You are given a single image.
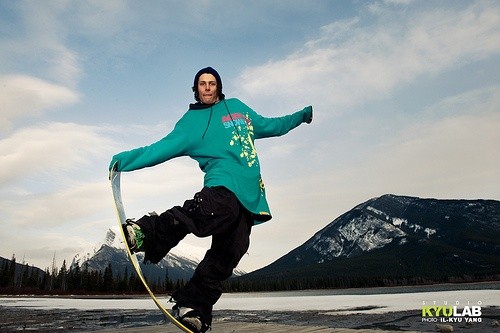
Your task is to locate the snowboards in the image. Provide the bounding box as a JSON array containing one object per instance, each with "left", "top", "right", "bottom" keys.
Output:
[{"left": 110, "top": 161, "right": 195, "bottom": 333}]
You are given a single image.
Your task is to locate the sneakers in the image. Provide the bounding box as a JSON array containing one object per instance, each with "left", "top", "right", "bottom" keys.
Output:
[
  {"left": 172, "top": 305, "right": 202, "bottom": 332},
  {"left": 121, "top": 218, "right": 146, "bottom": 255}
]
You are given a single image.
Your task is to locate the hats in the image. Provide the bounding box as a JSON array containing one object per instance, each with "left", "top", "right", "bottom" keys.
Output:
[{"left": 192, "top": 66, "right": 222, "bottom": 102}]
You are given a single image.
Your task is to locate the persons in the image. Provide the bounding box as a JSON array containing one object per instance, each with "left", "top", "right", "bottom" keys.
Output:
[{"left": 108, "top": 66, "right": 313, "bottom": 332}]
